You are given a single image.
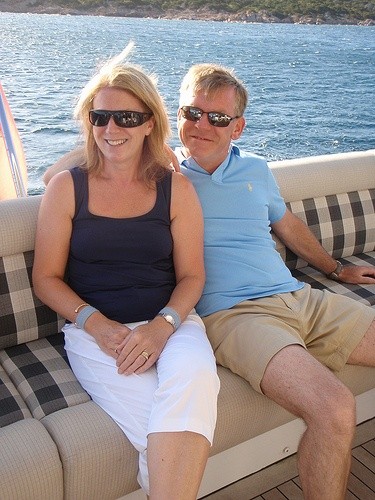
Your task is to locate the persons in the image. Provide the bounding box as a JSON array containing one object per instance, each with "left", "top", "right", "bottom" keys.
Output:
[
  {"left": 43, "top": 62, "right": 375, "bottom": 500},
  {"left": 33, "top": 65, "right": 220, "bottom": 500}
]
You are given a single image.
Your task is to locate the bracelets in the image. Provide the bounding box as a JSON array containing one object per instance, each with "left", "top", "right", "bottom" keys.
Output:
[
  {"left": 72, "top": 303, "right": 98, "bottom": 328},
  {"left": 159, "top": 306, "right": 181, "bottom": 330}
]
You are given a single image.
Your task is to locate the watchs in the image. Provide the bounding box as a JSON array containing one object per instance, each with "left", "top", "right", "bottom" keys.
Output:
[
  {"left": 157, "top": 312, "right": 176, "bottom": 332},
  {"left": 330, "top": 259, "right": 342, "bottom": 278}
]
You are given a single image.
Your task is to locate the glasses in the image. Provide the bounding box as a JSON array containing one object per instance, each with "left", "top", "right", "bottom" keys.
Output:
[
  {"left": 89, "top": 110, "right": 154, "bottom": 128},
  {"left": 181, "top": 106, "right": 242, "bottom": 127}
]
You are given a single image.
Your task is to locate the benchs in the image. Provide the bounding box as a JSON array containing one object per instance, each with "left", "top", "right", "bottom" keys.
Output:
[{"left": 0, "top": 148, "right": 375, "bottom": 500}]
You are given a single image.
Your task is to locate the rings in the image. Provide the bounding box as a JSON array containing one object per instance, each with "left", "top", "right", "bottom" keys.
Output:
[
  {"left": 141, "top": 351, "right": 148, "bottom": 359},
  {"left": 115, "top": 345, "right": 120, "bottom": 352}
]
